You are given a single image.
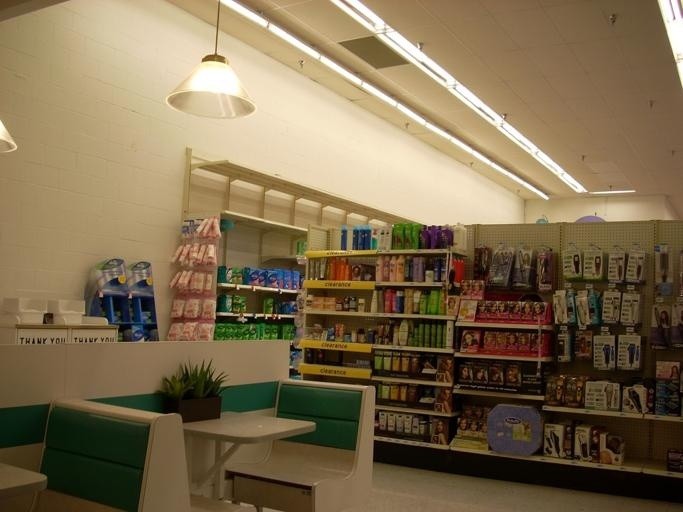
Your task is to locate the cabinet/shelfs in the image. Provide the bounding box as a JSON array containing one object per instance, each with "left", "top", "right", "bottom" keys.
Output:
[
  {"left": 183, "top": 147, "right": 424, "bottom": 343},
  {"left": 297, "top": 225, "right": 682, "bottom": 483}
]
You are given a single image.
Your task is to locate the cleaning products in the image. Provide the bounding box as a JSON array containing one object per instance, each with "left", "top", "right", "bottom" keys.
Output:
[{"left": 304, "top": 222, "right": 468, "bottom": 438}]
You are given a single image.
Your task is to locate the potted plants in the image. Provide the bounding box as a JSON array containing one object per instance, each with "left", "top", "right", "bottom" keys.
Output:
[{"left": 153, "top": 357, "right": 229, "bottom": 423}]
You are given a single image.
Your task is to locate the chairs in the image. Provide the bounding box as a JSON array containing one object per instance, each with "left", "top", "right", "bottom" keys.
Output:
[{"left": 30, "top": 378, "right": 376, "bottom": 511}]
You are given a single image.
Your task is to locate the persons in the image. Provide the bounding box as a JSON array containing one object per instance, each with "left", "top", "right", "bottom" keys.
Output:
[
  {"left": 428, "top": 281, "right": 544, "bottom": 445},
  {"left": 668, "top": 365, "right": 679, "bottom": 379},
  {"left": 660, "top": 310, "right": 669, "bottom": 326}
]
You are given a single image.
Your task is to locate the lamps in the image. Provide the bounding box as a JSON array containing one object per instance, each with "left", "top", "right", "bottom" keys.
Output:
[{"left": 164, "top": 0, "right": 256, "bottom": 120}]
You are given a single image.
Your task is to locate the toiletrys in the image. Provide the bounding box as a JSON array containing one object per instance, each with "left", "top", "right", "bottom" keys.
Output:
[
  {"left": 412, "top": 323, "right": 448, "bottom": 348},
  {"left": 303, "top": 294, "right": 399, "bottom": 346}
]
